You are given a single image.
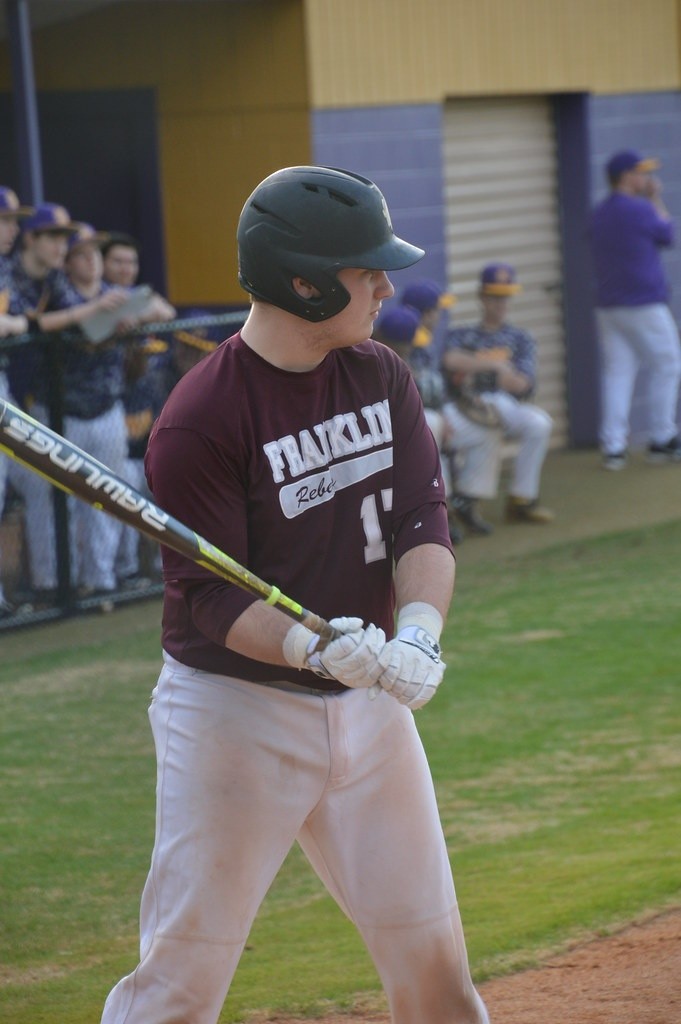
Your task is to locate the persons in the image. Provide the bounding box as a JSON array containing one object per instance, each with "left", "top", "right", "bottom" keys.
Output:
[
  {"left": 0, "top": 186, "right": 218, "bottom": 619},
  {"left": 441, "top": 263, "right": 554, "bottom": 534},
  {"left": 584, "top": 152, "right": 681, "bottom": 472},
  {"left": 97, "top": 166, "right": 491, "bottom": 1023},
  {"left": 375, "top": 263, "right": 500, "bottom": 543}
]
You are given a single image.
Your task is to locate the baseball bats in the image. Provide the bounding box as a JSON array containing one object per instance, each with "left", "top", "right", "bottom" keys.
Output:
[{"left": 0, "top": 398, "right": 342, "bottom": 643}]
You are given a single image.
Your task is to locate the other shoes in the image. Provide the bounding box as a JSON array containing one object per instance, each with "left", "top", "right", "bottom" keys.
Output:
[
  {"left": 447, "top": 494, "right": 495, "bottom": 535},
  {"left": 0, "top": 570, "right": 151, "bottom": 615},
  {"left": 647, "top": 437, "right": 681, "bottom": 461},
  {"left": 604, "top": 448, "right": 630, "bottom": 470},
  {"left": 449, "top": 526, "right": 465, "bottom": 545},
  {"left": 505, "top": 494, "right": 552, "bottom": 523}
]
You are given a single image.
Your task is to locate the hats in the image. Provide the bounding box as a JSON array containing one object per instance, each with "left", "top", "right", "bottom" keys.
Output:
[
  {"left": 70, "top": 221, "right": 108, "bottom": 249},
  {"left": 400, "top": 279, "right": 458, "bottom": 315},
  {"left": 478, "top": 263, "right": 522, "bottom": 297},
  {"left": 374, "top": 305, "right": 433, "bottom": 348},
  {"left": 0, "top": 188, "right": 36, "bottom": 220},
  {"left": 16, "top": 201, "right": 81, "bottom": 234}
]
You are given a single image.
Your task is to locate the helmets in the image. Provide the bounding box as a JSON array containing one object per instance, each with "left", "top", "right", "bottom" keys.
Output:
[{"left": 234, "top": 165, "right": 426, "bottom": 322}]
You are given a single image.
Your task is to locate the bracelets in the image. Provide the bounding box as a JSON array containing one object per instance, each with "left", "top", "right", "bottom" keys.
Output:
[
  {"left": 398, "top": 602, "right": 443, "bottom": 643},
  {"left": 283, "top": 623, "right": 316, "bottom": 668}
]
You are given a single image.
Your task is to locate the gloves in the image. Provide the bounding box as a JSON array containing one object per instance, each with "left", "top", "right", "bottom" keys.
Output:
[
  {"left": 368, "top": 625, "right": 447, "bottom": 710},
  {"left": 307, "top": 616, "right": 393, "bottom": 689}
]
